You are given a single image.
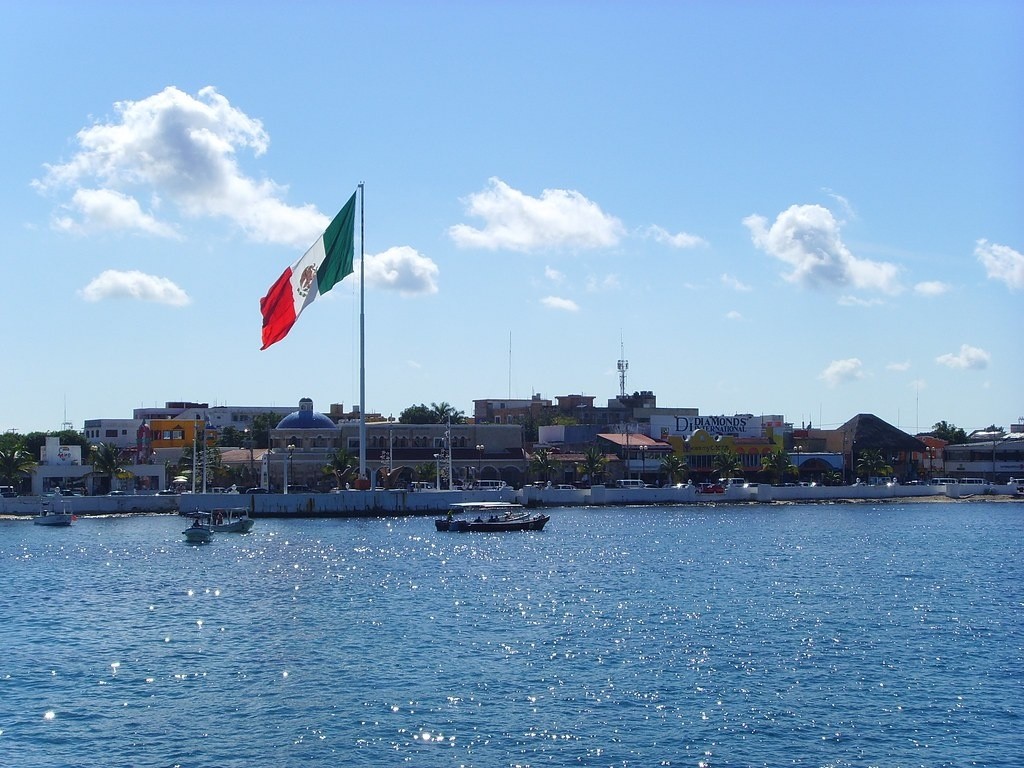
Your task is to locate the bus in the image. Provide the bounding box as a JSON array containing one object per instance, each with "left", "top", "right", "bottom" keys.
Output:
[
  {"left": 475, "top": 479, "right": 504, "bottom": 490},
  {"left": 930, "top": 477, "right": 958, "bottom": 485},
  {"left": 615, "top": 480, "right": 645, "bottom": 489},
  {"left": 960, "top": 477, "right": 988, "bottom": 485},
  {"left": 1014, "top": 479, "right": 1024, "bottom": 493},
  {"left": 717, "top": 478, "right": 744, "bottom": 488}
]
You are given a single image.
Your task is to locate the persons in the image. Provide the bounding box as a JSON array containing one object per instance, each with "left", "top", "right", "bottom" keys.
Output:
[
  {"left": 206, "top": 510, "right": 224, "bottom": 524},
  {"left": 461, "top": 481, "right": 478, "bottom": 490},
  {"left": 193, "top": 520, "right": 200, "bottom": 527},
  {"left": 416, "top": 481, "right": 421, "bottom": 492},
  {"left": 81, "top": 486, "right": 89, "bottom": 496},
  {"left": 494, "top": 515, "right": 500, "bottom": 522},
  {"left": 488, "top": 516, "right": 495, "bottom": 522},
  {"left": 446, "top": 508, "right": 453, "bottom": 522},
  {"left": 360, "top": 474, "right": 368, "bottom": 494},
  {"left": 474, "top": 516, "right": 484, "bottom": 523},
  {"left": 407, "top": 481, "right": 411, "bottom": 493}
]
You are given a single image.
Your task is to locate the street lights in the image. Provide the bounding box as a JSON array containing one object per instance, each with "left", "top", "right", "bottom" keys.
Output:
[
  {"left": 639, "top": 445, "right": 648, "bottom": 483},
  {"left": 925, "top": 446, "right": 936, "bottom": 485},
  {"left": 287, "top": 444, "right": 295, "bottom": 486},
  {"left": 794, "top": 446, "right": 803, "bottom": 485},
  {"left": 90, "top": 445, "right": 99, "bottom": 495},
  {"left": 475, "top": 444, "right": 484, "bottom": 479}
]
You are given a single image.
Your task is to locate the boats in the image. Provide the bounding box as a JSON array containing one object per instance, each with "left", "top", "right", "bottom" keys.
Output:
[
  {"left": 181, "top": 505, "right": 215, "bottom": 542},
  {"left": 434, "top": 500, "right": 552, "bottom": 531},
  {"left": 34, "top": 496, "right": 73, "bottom": 526},
  {"left": 210, "top": 507, "right": 255, "bottom": 531}
]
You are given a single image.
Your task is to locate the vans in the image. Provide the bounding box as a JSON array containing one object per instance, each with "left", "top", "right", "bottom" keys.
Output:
[
  {"left": 556, "top": 484, "right": 578, "bottom": 490},
  {"left": 0, "top": 486, "right": 14, "bottom": 498},
  {"left": 410, "top": 482, "right": 436, "bottom": 492}
]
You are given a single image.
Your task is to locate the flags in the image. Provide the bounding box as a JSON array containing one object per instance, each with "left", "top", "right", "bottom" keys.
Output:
[{"left": 258, "top": 190, "right": 356, "bottom": 350}]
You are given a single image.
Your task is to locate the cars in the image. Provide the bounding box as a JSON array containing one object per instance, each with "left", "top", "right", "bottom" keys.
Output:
[
  {"left": 662, "top": 483, "right": 725, "bottom": 494},
  {"left": 743, "top": 482, "right": 809, "bottom": 488},
  {"left": 107, "top": 491, "right": 125, "bottom": 495},
  {"left": 60, "top": 490, "right": 74, "bottom": 496},
  {"left": 640, "top": 484, "right": 660, "bottom": 488},
  {"left": 210, "top": 486, "right": 280, "bottom": 493},
  {"left": 155, "top": 490, "right": 177, "bottom": 495}
]
